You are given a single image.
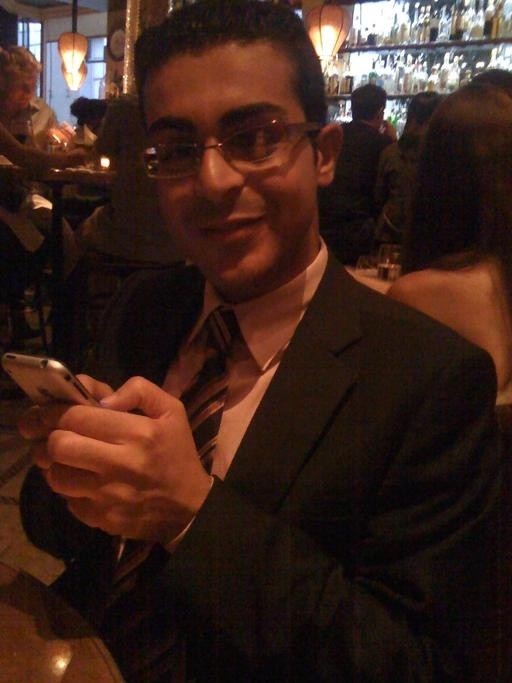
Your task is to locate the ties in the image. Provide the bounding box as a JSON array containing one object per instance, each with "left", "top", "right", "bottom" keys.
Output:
[{"left": 98, "top": 308, "right": 239, "bottom": 682}]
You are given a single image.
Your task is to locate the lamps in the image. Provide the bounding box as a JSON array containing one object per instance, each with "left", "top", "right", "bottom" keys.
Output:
[
  {"left": 305, "top": 3, "right": 353, "bottom": 73},
  {"left": 58, "top": 1, "right": 87, "bottom": 92}
]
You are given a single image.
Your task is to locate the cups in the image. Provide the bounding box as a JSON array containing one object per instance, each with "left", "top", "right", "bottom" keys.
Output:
[{"left": 376, "top": 243, "right": 402, "bottom": 280}]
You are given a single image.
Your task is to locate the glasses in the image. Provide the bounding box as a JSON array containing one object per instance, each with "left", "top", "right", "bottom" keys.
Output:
[{"left": 142, "top": 120, "right": 321, "bottom": 181}]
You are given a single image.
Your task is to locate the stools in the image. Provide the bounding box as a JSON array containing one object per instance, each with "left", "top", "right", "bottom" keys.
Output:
[{"left": 0, "top": 249, "right": 183, "bottom": 398}]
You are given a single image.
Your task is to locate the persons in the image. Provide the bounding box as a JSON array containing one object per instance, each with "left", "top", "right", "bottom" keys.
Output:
[
  {"left": 375, "top": 89, "right": 444, "bottom": 244},
  {"left": 384, "top": 80, "right": 512, "bottom": 431},
  {"left": 0, "top": 120, "right": 92, "bottom": 170},
  {"left": 468, "top": 68, "right": 512, "bottom": 100},
  {"left": 75, "top": 90, "right": 189, "bottom": 264},
  {"left": 18, "top": 0, "right": 506, "bottom": 681},
  {"left": 0, "top": 42, "right": 81, "bottom": 270},
  {"left": 318, "top": 83, "right": 399, "bottom": 264}
]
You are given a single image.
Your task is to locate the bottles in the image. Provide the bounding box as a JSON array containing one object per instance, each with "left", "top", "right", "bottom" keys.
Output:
[{"left": 332, "top": 0, "right": 512, "bottom": 137}]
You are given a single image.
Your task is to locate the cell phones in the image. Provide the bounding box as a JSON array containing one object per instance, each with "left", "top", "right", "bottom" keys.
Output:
[{"left": 1, "top": 351, "right": 104, "bottom": 407}]
